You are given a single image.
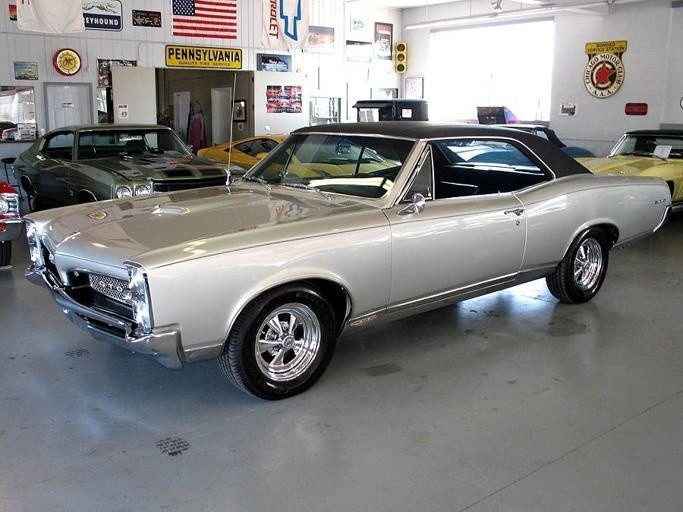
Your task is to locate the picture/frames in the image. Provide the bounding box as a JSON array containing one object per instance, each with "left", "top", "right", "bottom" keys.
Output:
[
  {"left": 349, "top": 15, "right": 366, "bottom": 34},
  {"left": 231, "top": 98, "right": 247, "bottom": 124},
  {"left": 404, "top": 78, "right": 424, "bottom": 98},
  {"left": 302, "top": 25, "right": 335, "bottom": 54},
  {"left": 373, "top": 21, "right": 394, "bottom": 60}
]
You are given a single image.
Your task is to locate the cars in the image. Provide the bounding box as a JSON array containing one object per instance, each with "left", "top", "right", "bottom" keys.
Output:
[
  {"left": 495, "top": 123, "right": 597, "bottom": 159},
  {"left": 1, "top": 178, "right": 21, "bottom": 265},
  {"left": 13, "top": 125, "right": 248, "bottom": 210},
  {"left": 573, "top": 129, "right": 681, "bottom": 213},
  {"left": 23, "top": 123, "right": 670, "bottom": 400}
]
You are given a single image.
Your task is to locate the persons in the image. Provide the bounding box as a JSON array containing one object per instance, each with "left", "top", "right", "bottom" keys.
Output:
[{"left": 185, "top": 100, "right": 208, "bottom": 157}]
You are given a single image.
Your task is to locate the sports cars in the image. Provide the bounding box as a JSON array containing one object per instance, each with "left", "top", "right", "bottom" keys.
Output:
[{"left": 198, "top": 133, "right": 402, "bottom": 179}]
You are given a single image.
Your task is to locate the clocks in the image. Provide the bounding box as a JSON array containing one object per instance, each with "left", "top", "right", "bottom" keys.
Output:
[{"left": 53, "top": 48, "right": 83, "bottom": 77}]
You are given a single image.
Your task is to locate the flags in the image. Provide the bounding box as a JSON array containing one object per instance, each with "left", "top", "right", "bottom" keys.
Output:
[
  {"left": 260, "top": 0, "right": 311, "bottom": 51},
  {"left": 169, "top": 0, "right": 239, "bottom": 40},
  {"left": 15, "top": 0, "right": 86, "bottom": 35}
]
